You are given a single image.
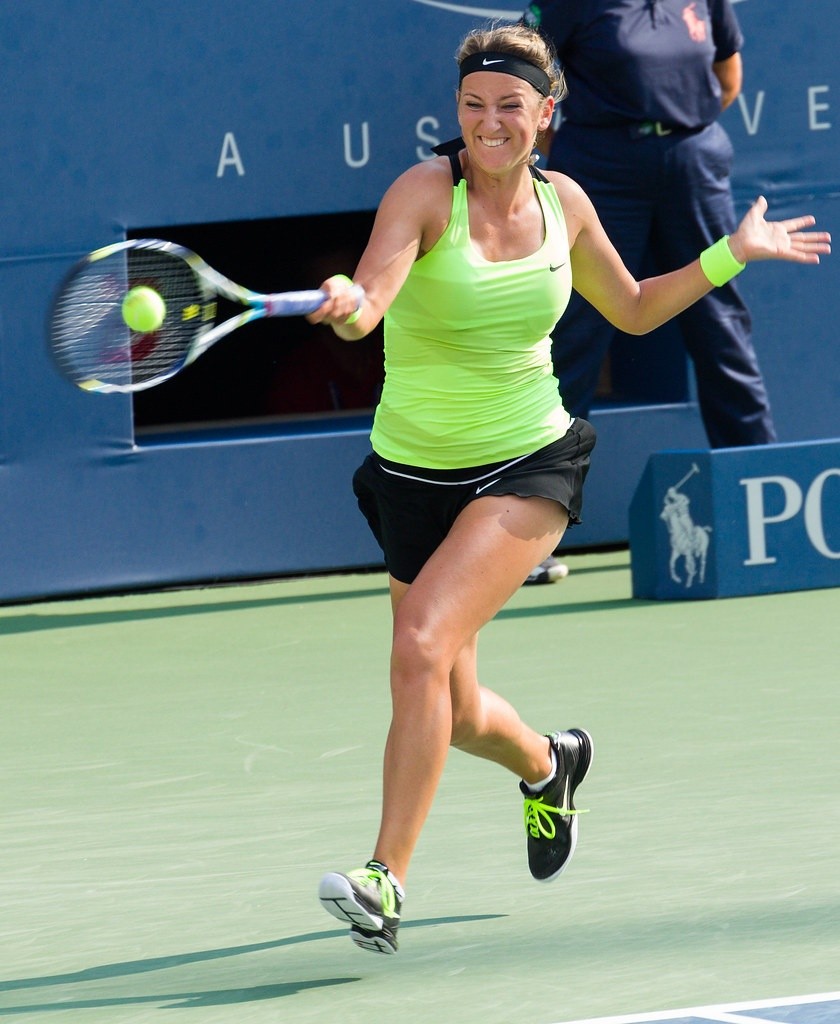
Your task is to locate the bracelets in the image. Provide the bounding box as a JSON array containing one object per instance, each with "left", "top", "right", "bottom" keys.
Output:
[
  {"left": 334, "top": 274, "right": 363, "bottom": 323},
  {"left": 700, "top": 235, "right": 747, "bottom": 286}
]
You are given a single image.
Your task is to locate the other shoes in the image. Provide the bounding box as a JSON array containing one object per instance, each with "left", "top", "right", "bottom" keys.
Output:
[{"left": 524, "top": 553, "right": 568, "bottom": 586}]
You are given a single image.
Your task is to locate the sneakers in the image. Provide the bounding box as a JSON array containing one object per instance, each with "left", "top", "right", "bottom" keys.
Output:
[
  {"left": 319, "top": 860, "right": 405, "bottom": 955},
  {"left": 520, "top": 727, "right": 593, "bottom": 882}
]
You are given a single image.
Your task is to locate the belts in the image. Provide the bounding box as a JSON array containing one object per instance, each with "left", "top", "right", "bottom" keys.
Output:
[{"left": 569, "top": 113, "right": 673, "bottom": 137}]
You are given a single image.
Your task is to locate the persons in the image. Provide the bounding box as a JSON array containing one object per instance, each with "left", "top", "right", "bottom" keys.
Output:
[
  {"left": 517, "top": 0, "right": 779, "bottom": 584},
  {"left": 311, "top": 25, "right": 832, "bottom": 954}
]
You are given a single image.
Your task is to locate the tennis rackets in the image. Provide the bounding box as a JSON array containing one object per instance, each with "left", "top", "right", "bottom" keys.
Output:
[{"left": 40, "top": 234, "right": 368, "bottom": 398}]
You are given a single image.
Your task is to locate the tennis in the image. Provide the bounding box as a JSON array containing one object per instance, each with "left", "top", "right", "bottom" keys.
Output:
[{"left": 120, "top": 284, "right": 167, "bottom": 334}]
